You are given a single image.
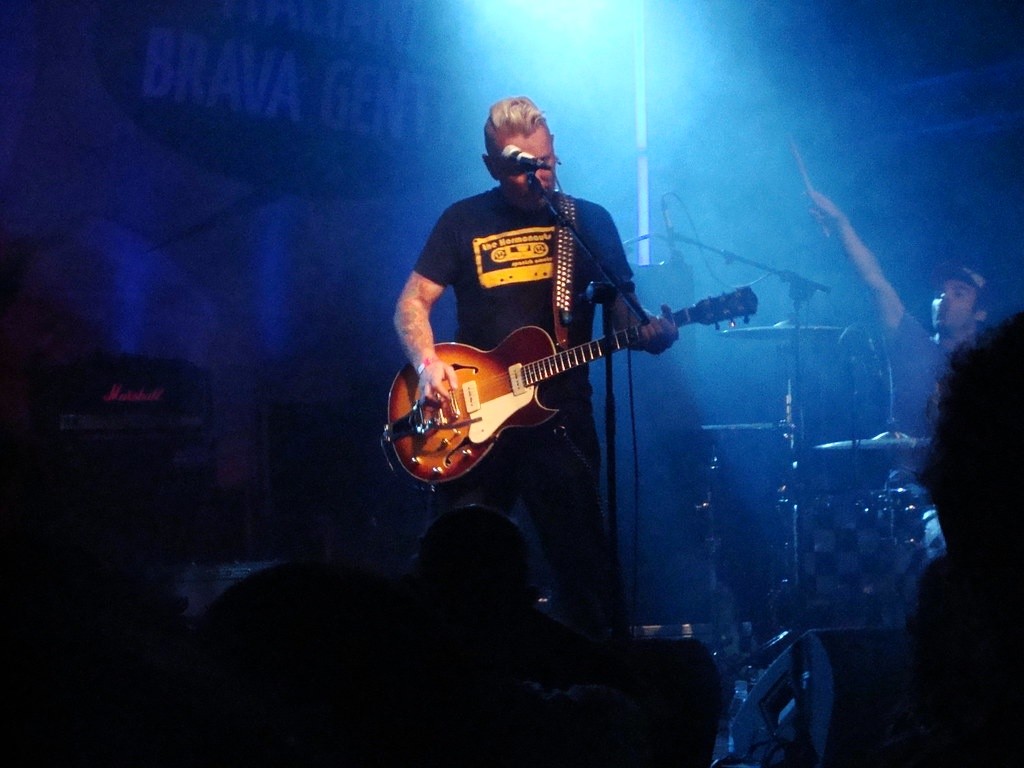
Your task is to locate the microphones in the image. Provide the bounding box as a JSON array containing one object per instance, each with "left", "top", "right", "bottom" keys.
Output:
[{"left": 500, "top": 144, "right": 553, "bottom": 171}]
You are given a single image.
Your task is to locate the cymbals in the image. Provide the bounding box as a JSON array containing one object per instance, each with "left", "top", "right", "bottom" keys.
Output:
[
  {"left": 722, "top": 316, "right": 845, "bottom": 340},
  {"left": 704, "top": 422, "right": 772, "bottom": 430},
  {"left": 812, "top": 431, "right": 931, "bottom": 449}
]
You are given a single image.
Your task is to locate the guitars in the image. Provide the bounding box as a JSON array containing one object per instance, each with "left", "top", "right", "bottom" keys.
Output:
[{"left": 388, "top": 287, "right": 759, "bottom": 482}]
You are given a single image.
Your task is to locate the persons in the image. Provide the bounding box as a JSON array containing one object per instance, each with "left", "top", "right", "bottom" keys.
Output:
[
  {"left": 0, "top": 241, "right": 719, "bottom": 768},
  {"left": 729, "top": 126, "right": 1024, "bottom": 767},
  {"left": 392, "top": 96, "right": 678, "bottom": 643}
]
